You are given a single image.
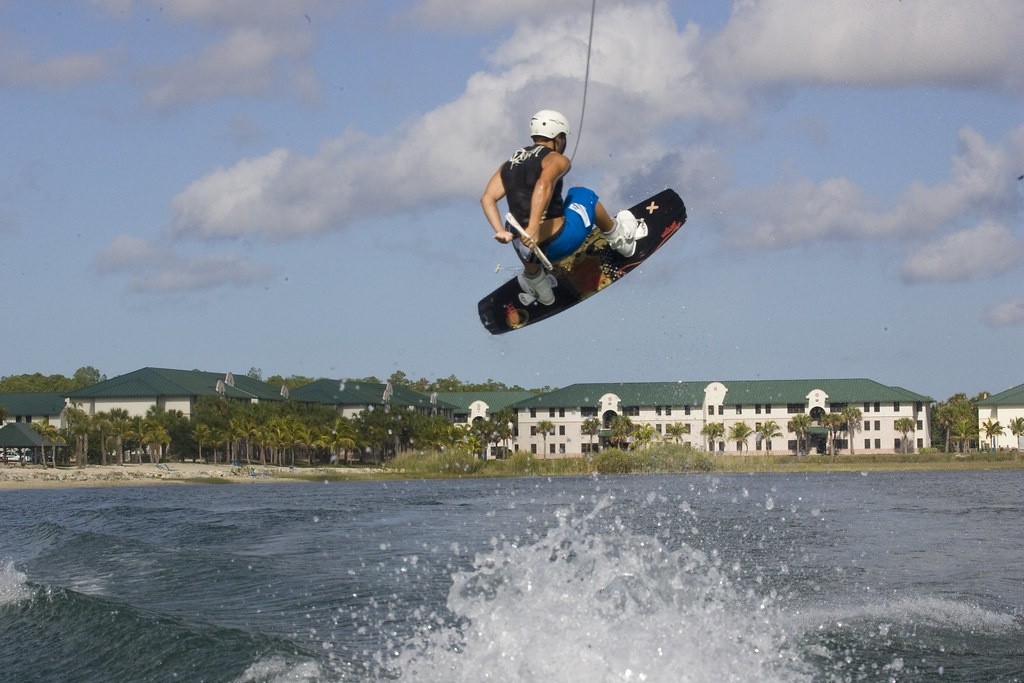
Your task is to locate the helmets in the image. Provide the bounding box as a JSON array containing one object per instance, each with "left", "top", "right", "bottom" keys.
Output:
[{"left": 529, "top": 109, "right": 570, "bottom": 139}]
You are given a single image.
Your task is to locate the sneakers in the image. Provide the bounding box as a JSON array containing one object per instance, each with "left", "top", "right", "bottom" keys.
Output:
[
  {"left": 607, "top": 209, "right": 639, "bottom": 258},
  {"left": 517, "top": 262, "right": 556, "bottom": 306}
]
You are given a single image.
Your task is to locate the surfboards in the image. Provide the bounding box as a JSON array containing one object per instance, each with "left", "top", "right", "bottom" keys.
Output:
[{"left": 476, "top": 187, "right": 688, "bottom": 337}]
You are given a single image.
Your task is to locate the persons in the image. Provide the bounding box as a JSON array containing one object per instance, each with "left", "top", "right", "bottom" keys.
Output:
[{"left": 480, "top": 108, "right": 638, "bottom": 306}]
